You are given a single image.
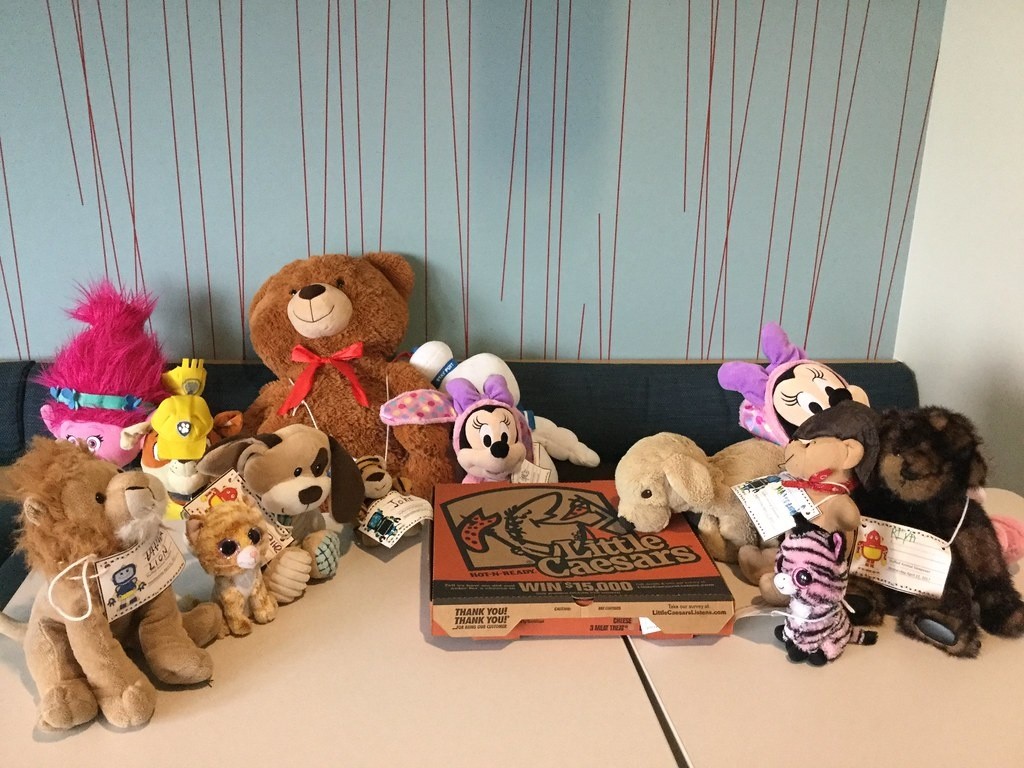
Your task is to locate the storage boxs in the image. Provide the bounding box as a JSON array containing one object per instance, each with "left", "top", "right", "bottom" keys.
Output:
[{"left": 429, "top": 481, "right": 734, "bottom": 641}]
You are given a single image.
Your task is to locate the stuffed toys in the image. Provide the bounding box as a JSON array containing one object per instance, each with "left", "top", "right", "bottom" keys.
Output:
[
  {"left": 615, "top": 322, "right": 1024, "bottom": 668},
  {"left": 0, "top": 252, "right": 601, "bottom": 730}
]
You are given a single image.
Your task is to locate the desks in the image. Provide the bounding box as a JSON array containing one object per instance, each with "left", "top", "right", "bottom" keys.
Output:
[
  {"left": 0, "top": 535, "right": 684, "bottom": 767},
  {"left": 629, "top": 551, "right": 1024, "bottom": 768}
]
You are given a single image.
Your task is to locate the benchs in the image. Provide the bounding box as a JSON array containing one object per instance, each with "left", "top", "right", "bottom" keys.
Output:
[{"left": 0, "top": 360, "right": 923, "bottom": 612}]
